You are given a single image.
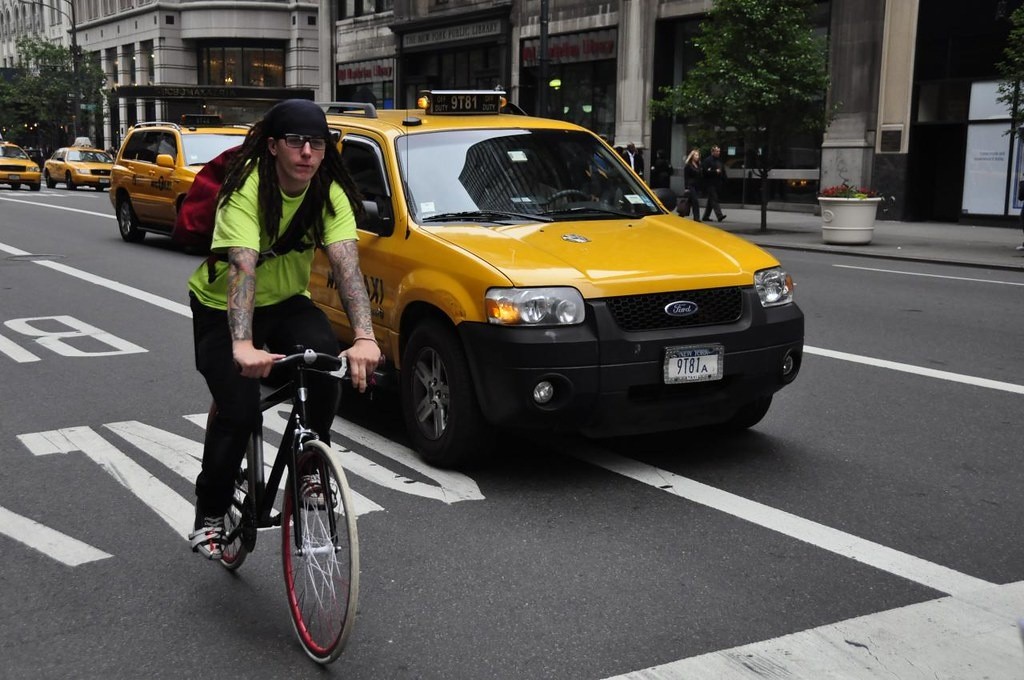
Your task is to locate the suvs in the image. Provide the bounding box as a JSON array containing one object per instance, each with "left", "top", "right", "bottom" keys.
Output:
[
  {"left": 294, "top": 89, "right": 807, "bottom": 470},
  {"left": 107, "top": 114, "right": 258, "bottom": 244}
]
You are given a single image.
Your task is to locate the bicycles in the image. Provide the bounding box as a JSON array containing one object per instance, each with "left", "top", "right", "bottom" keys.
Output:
[{"left": 186, "top": 342, "right": 392, "bottom": 665}]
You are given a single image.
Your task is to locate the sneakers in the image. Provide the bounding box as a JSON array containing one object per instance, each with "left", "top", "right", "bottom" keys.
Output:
[
  {"left": 298, "top": 476, "right": 336, "bottom": 510},
  {"left": 193, "top": 497, "right": 227, "bottom": 560}
]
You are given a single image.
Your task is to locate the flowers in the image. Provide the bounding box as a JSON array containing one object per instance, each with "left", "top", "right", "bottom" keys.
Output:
[{"left": 816, "top": 183, "right": 886, "bottom": 198}]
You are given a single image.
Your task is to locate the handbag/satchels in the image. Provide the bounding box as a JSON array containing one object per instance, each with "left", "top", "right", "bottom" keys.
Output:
[{"left": 168, "top": 137, "right": 334, "bottom": 259}]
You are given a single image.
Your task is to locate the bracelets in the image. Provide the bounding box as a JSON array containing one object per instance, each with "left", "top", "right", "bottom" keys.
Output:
[{"left": 352, "top": 337, "right": 379, "bottom": 347}]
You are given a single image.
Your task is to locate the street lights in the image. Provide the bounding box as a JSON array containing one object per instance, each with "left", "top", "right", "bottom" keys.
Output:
[{"left": 17, "top": 0, "right": 82, "bottom": 135}]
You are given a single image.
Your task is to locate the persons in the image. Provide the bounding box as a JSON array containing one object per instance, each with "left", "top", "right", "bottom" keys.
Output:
[
  {"left": 654, "top": 149, "right": 674, "bottom": 189},
  {"left": 186, "top": 100, "right": 382, "bottom": 559},
  {"left": 592, "top": 133, "right": 644, "bottom": 211},
  {"left": 678, "top": 145, "right": 727, "bottom": 223}
]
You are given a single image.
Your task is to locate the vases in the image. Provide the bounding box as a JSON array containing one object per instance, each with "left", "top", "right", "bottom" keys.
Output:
[{"left": 818, "top": 197, "right": 882, "bottom": 245}]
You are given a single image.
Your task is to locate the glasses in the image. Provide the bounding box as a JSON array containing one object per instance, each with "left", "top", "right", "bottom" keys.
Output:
[{"left": 276, "top": 134, "right": 328, "bottom": 150}]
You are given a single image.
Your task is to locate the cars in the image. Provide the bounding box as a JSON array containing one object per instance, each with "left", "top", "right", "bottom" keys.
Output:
[
  {"left": 0, "top": 140, "right": 42, "bottom": 192},
  {"left": 43, "top": 137, "right": 116, "bottom": 191}
]
